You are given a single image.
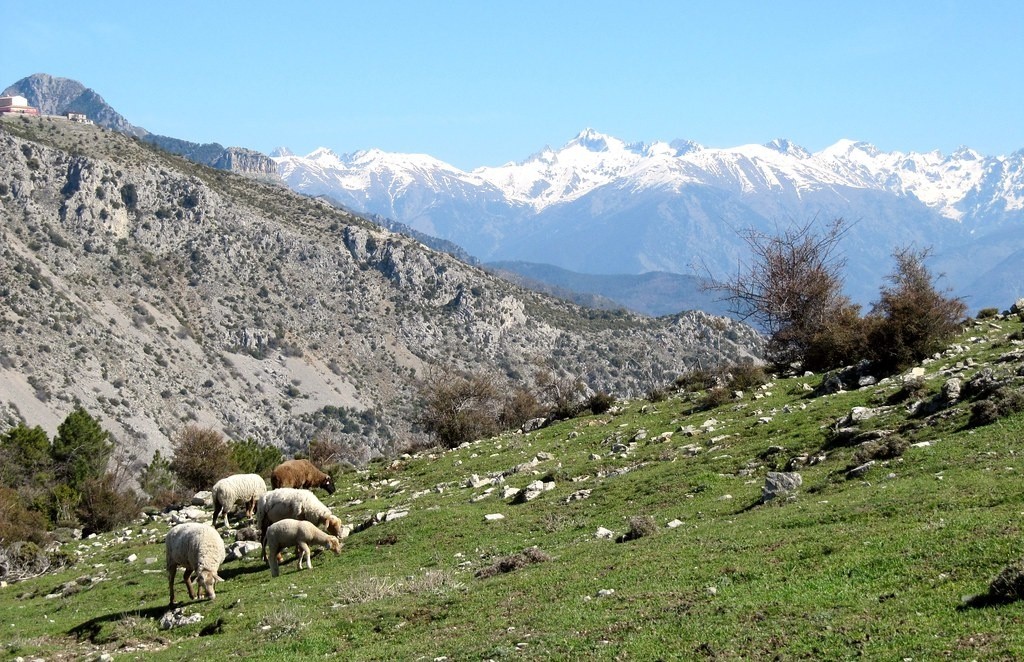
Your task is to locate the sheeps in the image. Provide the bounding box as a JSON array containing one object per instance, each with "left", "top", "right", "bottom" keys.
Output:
[
  {"left": 270, "top": 458, "right": 336, "bottom": 495},
  {"left": 166, "top": 522, "right": 226, "bottom": 606},
  {"left": 256, "top": 487, "right": 344, "bottom": 578},
  {"left": 211, "top": 473, "right": 268, "bottom": 529}
]
